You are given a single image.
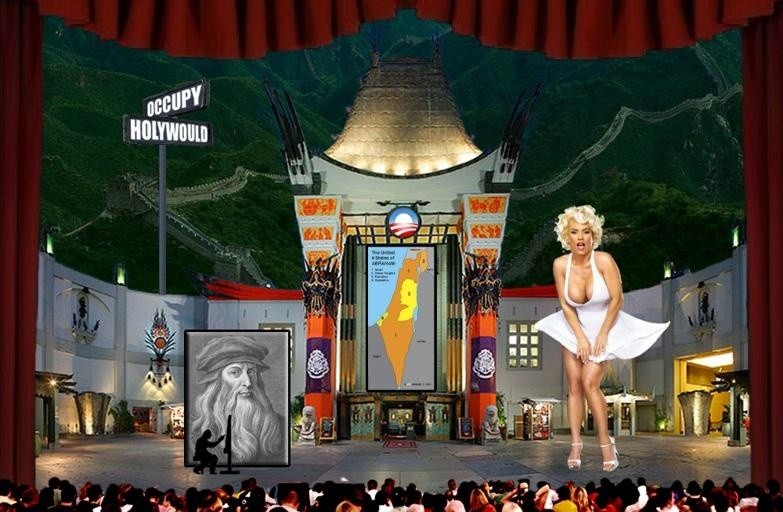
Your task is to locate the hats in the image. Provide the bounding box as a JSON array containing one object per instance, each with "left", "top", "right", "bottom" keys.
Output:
[{"left": 194, "top": 335, "right": 272, "bottom": 384}]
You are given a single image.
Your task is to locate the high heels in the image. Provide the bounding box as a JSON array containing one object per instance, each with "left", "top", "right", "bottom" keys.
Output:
[
  {"left": 600, "top": 436, "right": 620, "bottom": 473},
  {"left": 566, "top": 438, "right": 584, "bottom": 472}
]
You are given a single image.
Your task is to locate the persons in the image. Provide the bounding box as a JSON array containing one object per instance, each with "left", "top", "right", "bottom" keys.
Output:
[
  {"left": 532, "top": 203, "right": 671, "bottom": 472},
  {"left": 1, "top": 476, "right": 782, "bottom": 512},
  {"left": 192, "top": 334, "right": 285, "bottom": 464},
  {"left": 193, "top": 430, "right": 226, "bottom": 474}
]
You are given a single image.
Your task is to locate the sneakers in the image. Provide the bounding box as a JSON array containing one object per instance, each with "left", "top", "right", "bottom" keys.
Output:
[
  {"left": 209, "top": 472, "right": 219, "bottom": 475},
  {"left": 193, "top": 470, "right": 201, "bottom": 475}
]
